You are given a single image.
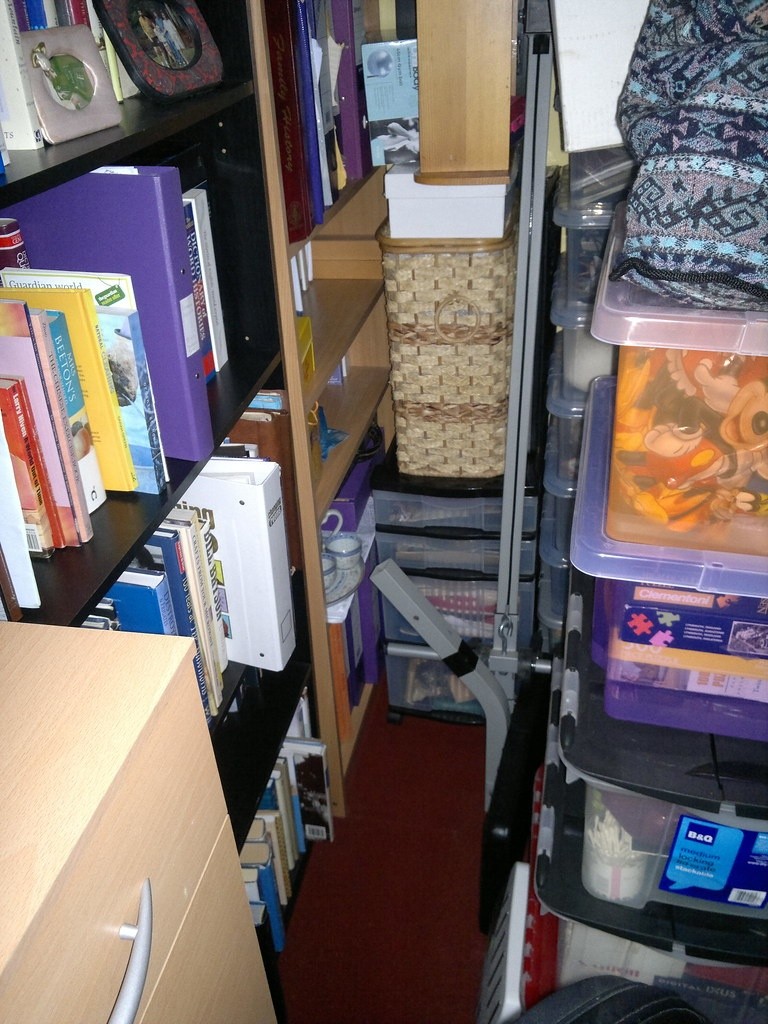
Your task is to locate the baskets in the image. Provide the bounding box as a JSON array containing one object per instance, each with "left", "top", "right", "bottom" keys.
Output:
[{"left": 375, "top": 187, "right": 522, "bottom": 478}]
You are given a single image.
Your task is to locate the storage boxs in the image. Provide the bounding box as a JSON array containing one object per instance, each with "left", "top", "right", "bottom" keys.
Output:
[{"left": 373, "top": 149, "right": 768, "bottom": 1024}]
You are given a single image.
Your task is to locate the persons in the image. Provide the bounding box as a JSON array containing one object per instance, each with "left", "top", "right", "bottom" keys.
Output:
[
  {"left": 45, "top": 62, "right": 79, "bottom": 109},
  {"left": 139, "top": 11, "right": 186, "bottom": 68}
]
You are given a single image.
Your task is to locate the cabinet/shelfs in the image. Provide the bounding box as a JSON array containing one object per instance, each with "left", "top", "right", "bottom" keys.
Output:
[{"left": 0, "top": 0, "right": 396, "bottom": 1024}]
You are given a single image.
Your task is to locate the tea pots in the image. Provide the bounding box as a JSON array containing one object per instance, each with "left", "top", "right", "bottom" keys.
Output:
[{"left": 320, "top": 509, "right": 343, "bottom": 553}]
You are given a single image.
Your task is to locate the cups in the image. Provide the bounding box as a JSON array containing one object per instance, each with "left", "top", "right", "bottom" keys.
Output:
[
  {"left": 325, "top": 533, "right": 362, "bottom": 570},
  {"left": 321, "top": 553, "right": 337, "bottom": 592}
]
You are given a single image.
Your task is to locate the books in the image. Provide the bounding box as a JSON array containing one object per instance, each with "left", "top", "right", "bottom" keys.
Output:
[
  {"left": 249, "top": 393, "right": 283, "bottom": 410},
  {"left": 0, "top": 165, "right": 228, "bottom": 610},
  {"left": 266, "top": 0, "right": 420, "bottom": 242},
  {"left": 291, "top": 241, "right": 314, "bottom": 310},
  {"left": 240, "top": 694, "right": 334, "bottom": 1024},
  {"left": 327, "top": 355, "right": 346, "bottom": 384},
  {"left": 0, "top": 0, "right": 142, "bottom": 174},
  {"left": 80, "top": 437, "right": 296, "bottom": 725}
]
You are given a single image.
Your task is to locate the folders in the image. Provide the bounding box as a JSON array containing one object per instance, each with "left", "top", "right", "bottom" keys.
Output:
[{"left": 0, "top": 165, "right": 214, "bottom": 463}]
